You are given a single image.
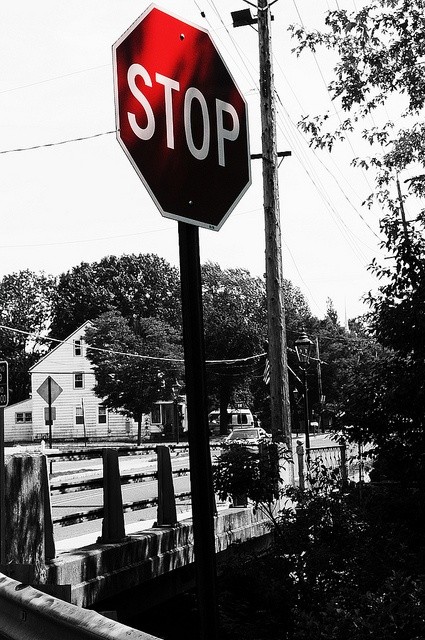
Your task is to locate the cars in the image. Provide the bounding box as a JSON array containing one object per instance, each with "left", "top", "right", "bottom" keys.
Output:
[{"left": 225, "top": 427, "right": 271, "bottom": 454}]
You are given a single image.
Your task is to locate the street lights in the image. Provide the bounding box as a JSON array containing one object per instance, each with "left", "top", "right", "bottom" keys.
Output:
[
  {"left": 170, "top": 378, "right": 182, "bottom": 444},
  {"left": 294, "top": 322, "right": 315, "bottom": 469}
]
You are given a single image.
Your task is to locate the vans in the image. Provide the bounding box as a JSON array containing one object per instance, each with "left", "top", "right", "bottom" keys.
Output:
[{"left": 208, "top": 408, "right": 255, "bottom": 429}]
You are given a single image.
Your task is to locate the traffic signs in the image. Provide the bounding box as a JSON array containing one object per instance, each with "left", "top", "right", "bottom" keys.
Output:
[{"left": 111, "top": 3, "right": 251, "bottom": 231}]
